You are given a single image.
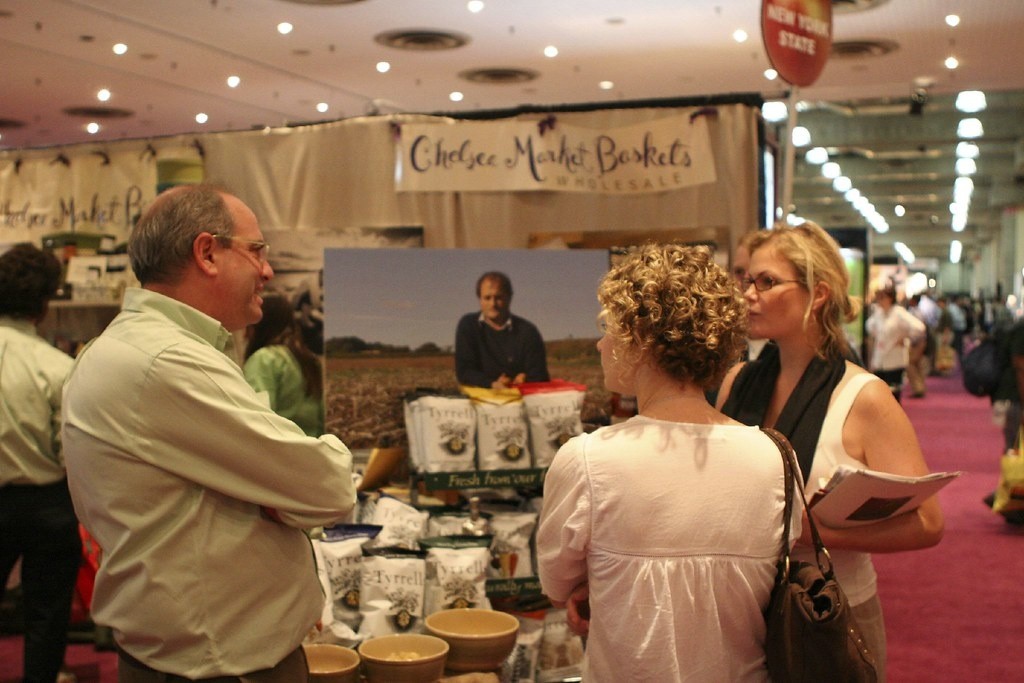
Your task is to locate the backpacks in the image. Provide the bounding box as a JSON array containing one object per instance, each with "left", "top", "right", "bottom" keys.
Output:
[{"left": 961, "top": 335, "right": 1010, "bottom": 396}]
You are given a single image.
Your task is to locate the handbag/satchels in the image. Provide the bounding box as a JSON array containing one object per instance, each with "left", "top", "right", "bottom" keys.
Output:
[
  {"left": 992, "top": 425, "right": 1024, "bottom": 512},
  {"left": 759, "top": 426, "right": 879, "bottom": 683},
  {"left": 936, "top": 344, "right": 956, "bottom": 373}
]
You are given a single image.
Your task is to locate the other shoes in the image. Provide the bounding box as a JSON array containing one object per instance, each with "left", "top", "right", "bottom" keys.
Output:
[
  {"left": 909, "top": 393, "right": 923, "bottom": 398},
  {"left": 984, "top": 491, "right": 1024, "bottom": 525}
]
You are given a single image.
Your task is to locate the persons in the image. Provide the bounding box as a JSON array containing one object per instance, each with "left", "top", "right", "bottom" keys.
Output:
[
  {"left": 454, "top": 271, "right": 551, "bottom": 388},
  {"left": 715, "top": 220, "right": 944, "bottom": 682},
  {"left": 59, "top": 181, "right": 358, "bottom": 683},
  {"left": 536, "top": 237, "right": 805, "bottom": 683},
  {"left": 0, "top": 240, "right": 75, "bottom": 683},
  {"left": 863, "top": 278, "right": 1024, "bottom": 526}
]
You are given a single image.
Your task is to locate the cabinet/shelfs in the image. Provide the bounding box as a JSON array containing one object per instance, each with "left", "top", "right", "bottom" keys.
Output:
[{"left": 411, "top": 466, "right": 546, "bottom": 597}]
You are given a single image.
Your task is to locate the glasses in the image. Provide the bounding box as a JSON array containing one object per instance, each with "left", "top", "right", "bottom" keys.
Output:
[
  {"left": 212, "top": 234, "right": 269, "bottom": 264},
  {"left": 743, "top": 275, "right": 802, "bottom": 291}
]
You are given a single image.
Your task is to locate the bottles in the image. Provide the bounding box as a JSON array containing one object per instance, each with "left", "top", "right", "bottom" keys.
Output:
[
  {"left": 45, "top": 239, "right": 60, "bottom": 300},
  {"left": 58, "top": 241, "right": 78, "bottom": 299}
]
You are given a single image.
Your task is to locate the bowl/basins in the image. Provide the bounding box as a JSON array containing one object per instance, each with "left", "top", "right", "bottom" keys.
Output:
[
  {"left": 304, "top": 644, "right": 362, "bottom": 683},
  {"left": 424, "top": 609, "right": 520, "bottom": 672},
  {"left": 359, "top": 633, "right": 450, "bottom": 683}
]
